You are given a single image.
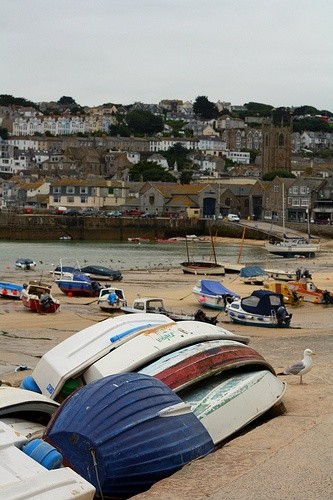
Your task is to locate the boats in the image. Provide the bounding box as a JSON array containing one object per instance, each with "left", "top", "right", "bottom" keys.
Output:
[
  {"left": 178, "top": 260, "right": 226, "bottom": 277},
  {"left": 261, "top": 238, "right": 321, "bottom": 259},
  {"left": 0, "top": 385, "right": 96, "bottom": 500},
  {"left": 19, "top": 310, "right": 288, "bottom": 445},
  {"left": 240, "top": 265, "right": 298, "bottom": 284},
  {"left": 42, "top": 371, "right": 217, "bottom": 500},
  {"left": 48, "top": 262, "right": 124, "bottom": 282},
  {"left": 192, "top": 238, "right": 211, "bottom": 243},
  {"left": 54, "top": 258, "right": 111, "bottom": 297},
  {"left": 225, "top": 289, "right": 293, "bottom": 329},
  {"left": 157, "top": 237, "right": 182, "bottom": 246},
  {"left": 127, "top": 236, "right": 158, "bottom": 244},
  {"left": 176, "top": 234, "right": 198, "bottom": 244},
  {"left": 20, "top": 269, "right": 61, "bottom": 315},
  {"left": 191, "top": 279, "right": 240, "bottom": 311},
  {"left": 219, "top": 262, "right": 246, "bottom": 273},
  {"left": 97, "top": 288, "right": 127, "bottom": 312},
  {"left": 0, "top": 280, "right": 24, "bottom": 298},
  {"left": 14, "top": 258, "right": 37, "bottom": 269},
  {"left": 119, "top": 296, "right": 195, "bottom": 321},
  {"left": 58, "top": 233, "right": 72, "bottom": 240},
  {"left": 287, "top": 281, "right": 332, "bottom": 305}
]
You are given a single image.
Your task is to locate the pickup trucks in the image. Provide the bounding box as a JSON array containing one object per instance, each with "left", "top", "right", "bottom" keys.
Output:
[{"left": 106, "top": 210, "right": 123, "bottom": 218}]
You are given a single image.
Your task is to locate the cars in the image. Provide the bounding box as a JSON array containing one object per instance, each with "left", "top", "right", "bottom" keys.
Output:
[
  {"left": 52, "top": 206, "right": 67, "bottom": 215},
  {"left": 78, "top": 208, "right": 95, "bottom": 216},
  {"left": 63, "top": 207, "right": 82, "bottom": 216},
  {"left": 141, "top": 212, "right": 159, "bottom": 219}
]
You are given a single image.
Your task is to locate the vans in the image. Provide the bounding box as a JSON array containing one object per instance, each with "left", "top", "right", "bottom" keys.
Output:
[{"left": 227, "top": 214, "right": 240, "bottom": 223}]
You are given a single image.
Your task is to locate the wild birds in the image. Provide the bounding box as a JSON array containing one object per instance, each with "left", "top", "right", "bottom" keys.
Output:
[{"left": 277, "top": 349, "right": 317, "bottom": 385}]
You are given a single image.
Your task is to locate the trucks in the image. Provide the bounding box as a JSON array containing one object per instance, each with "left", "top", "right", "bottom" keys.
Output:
[
  {"left": 127, "top": 209, "right": 140, "bottom": 216},
  {"left": 178, "top": 208, "right": 201, "bottom": 219}
]
